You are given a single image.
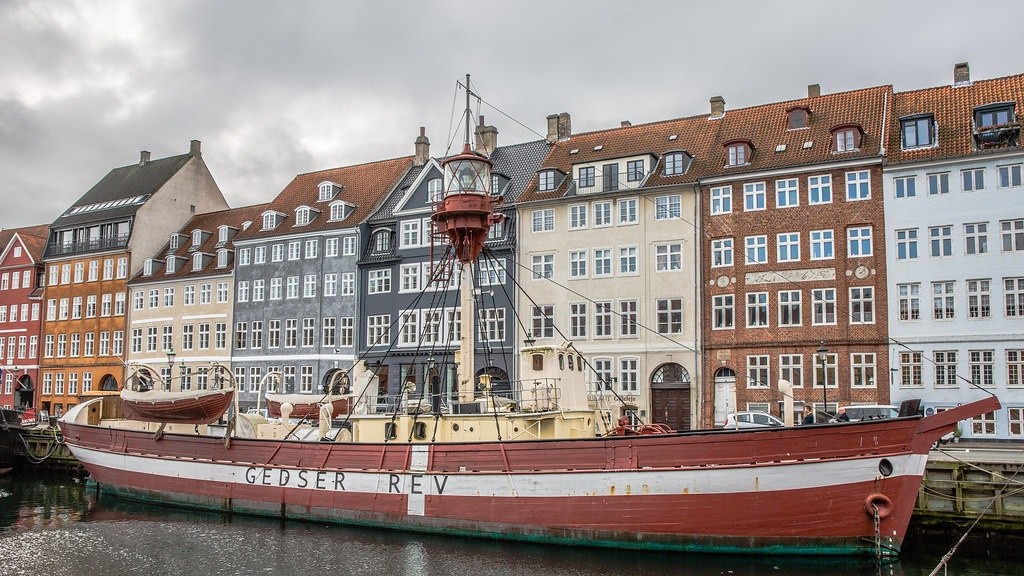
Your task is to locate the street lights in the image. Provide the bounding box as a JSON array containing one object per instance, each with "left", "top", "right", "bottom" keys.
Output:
[
  {"left": 166, "top": 346, "right": 177, "bottom": 394},
  {"left": 815, "top": 341, "right": 830, "bottom": 423}
]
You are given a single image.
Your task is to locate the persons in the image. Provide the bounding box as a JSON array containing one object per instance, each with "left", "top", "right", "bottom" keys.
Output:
[
  {"left": 831, "top": 407, "right": 850, "bottom": 424},
  {"left": 800, "top": 405, "right": 815, "bottom": 426}
]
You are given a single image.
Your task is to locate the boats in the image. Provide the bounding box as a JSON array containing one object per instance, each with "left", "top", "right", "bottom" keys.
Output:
[
  {"left": 264, "top": 391, "right": 359, "bottom": 421},
  {"left": 116, "top": 385, "right": 234, "bottom": 425},
  {"left": 55, "top": 75, "right": 1002, "bottom": 569}
]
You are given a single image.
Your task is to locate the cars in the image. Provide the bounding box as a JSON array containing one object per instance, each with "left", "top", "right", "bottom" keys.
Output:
[
  {"left": 721, "top": 410, "right": 784, "bottom": 432},
  {"left": 927, "top": 429, "right": 955, "bottom": 450},
  {"left": 836, "top": 405, "right": 900, "bottom": 422}
]
